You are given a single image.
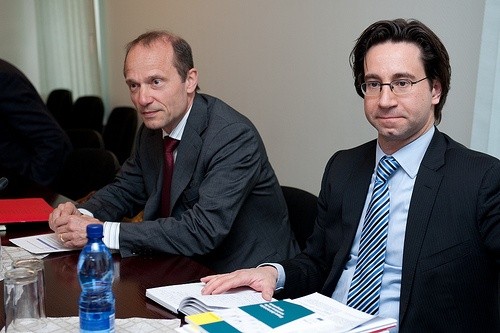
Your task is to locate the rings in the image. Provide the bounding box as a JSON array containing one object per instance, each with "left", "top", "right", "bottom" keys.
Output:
[{"left": 60, "top": 234, "right": 65, "bottom": 243}]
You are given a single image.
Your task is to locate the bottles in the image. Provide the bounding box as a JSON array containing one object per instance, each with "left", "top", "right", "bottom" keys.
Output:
[{"left": 77, "top": 224, "right": 115, "bottom": 333}]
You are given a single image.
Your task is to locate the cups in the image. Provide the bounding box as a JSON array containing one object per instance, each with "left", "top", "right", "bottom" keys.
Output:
[{"left": 4, "top": 257, "right": 47, "bottom": 333}]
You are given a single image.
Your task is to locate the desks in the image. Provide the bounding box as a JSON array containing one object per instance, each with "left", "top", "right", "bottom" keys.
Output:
[{"left": 0, "top": 222, "right": 299, "bottom": 333}]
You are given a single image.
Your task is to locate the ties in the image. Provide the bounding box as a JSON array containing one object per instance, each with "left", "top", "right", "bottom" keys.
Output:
[
  {"left": 158, "top": 137, "right": 179, "bottom": 219},
  {"left": 347, "top": 156, "right": 400, "bottom": 317}
]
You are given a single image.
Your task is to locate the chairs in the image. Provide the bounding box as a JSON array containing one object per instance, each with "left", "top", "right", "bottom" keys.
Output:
[
  {"left": 104, "top": 105, "right": 137, "bottom": 161},
  {"left": 47, "top": 89, "right": 74, "bottom": 126},
  {"left": 68, "top": 151, "right": 119, "bottom": 200},
  {"left": 66, "top": 96, "right": 107, "bottom": 131},
  {"left": 278, "top": 186, "right": 318, "bottom": 250},
  {"left": 66, "top": 128, "right": 105, "bottom": 155}
]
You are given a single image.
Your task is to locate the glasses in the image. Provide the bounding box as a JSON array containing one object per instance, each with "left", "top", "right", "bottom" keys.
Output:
[{"left": 360, "top": 78, "right": 426, "bottom": 96}]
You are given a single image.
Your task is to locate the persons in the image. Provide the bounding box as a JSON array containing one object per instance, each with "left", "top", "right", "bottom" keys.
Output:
[
  {"left": 49, "top": 32, "right": 302, "bottom": 273},
  {"left": 200, "top": 18, "right": 500, "bottom": 333},
  {"left": 0, "top": 60, "right": 73, "bottom": 186}
]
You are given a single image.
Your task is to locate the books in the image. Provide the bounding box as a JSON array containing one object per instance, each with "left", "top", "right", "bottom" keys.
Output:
[{"left": 145, "top": 282, "right": 398, "bottom": 333}]
